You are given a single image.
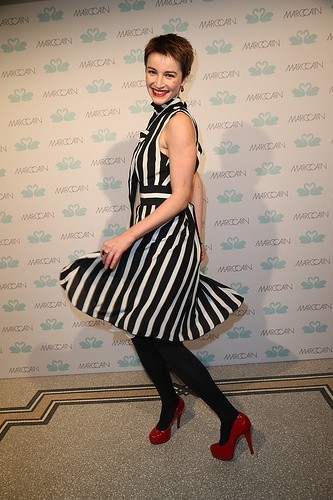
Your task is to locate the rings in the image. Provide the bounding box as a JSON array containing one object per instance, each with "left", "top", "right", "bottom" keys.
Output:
[{"left": 101, "top": 250, "right": 106, "bottom": 255}]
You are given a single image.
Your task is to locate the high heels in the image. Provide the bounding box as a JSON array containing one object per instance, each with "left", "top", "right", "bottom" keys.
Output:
[
  {"left": 148, "top": 397, "right": 185, "bottom": 446},
  {"left": 209, "top": 410, "right": 256, "bottom": 462}
]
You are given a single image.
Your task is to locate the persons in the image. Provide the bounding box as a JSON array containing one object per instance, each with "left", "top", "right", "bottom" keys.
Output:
[{"left": 60, "top": 33, "right": 256, "bottom": 462}]
